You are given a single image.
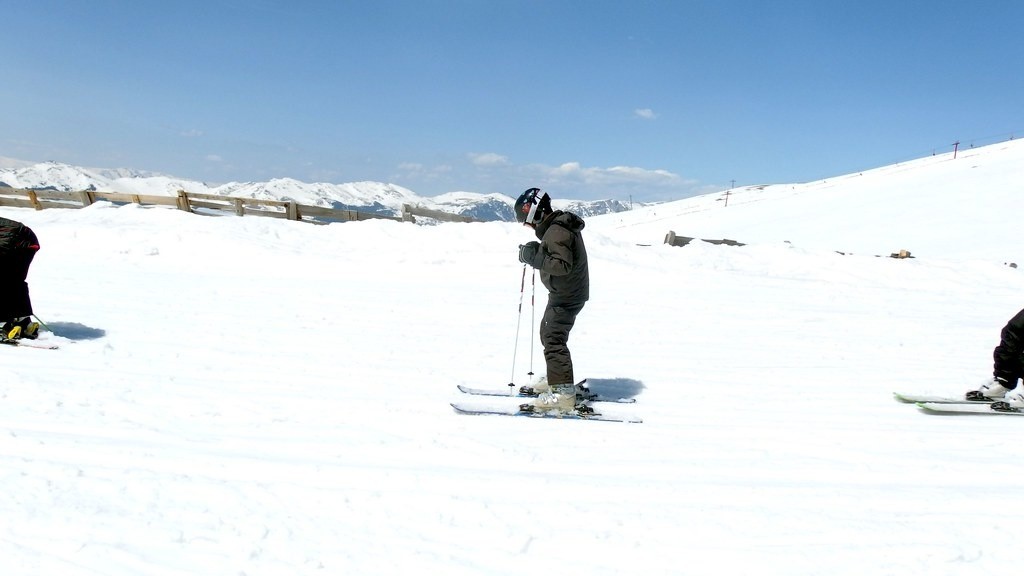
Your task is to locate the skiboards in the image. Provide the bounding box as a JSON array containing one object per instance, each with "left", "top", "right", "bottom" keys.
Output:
[
  {"left": 450, "top": 385, "right": 643, "bottom": 424},
  {"left": 892, "top": 391, "right": 1024, "bottom": 417},
  {"left": 1, "top": 336, "right": 78, "bottom": 350}
]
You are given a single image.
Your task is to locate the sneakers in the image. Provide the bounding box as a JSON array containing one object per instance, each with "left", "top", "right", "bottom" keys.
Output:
[
  {"left": 533, "top": 376, "right": 577, "bottom": 412},
  {"left": 966, "top": 380, "right": 1024, "bottom": 412},
  {"left": 0, "top": 316, "right": 39, "bottom": 344}
]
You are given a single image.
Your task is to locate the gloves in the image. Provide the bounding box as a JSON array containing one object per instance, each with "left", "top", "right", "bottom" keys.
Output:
[{"left": 518, "top": 241, "right": 545, "bottom": 270}]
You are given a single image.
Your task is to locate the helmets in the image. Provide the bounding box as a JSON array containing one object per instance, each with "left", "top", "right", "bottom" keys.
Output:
[{"left": 515, "top": 188, "right": 550, "bottom": 227}]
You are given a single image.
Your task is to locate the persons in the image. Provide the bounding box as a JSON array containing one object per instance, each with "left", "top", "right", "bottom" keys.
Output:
[
  {"left": 514, "top": 187, "right": 589, "bottom": 414},
  {"left": 965, "top": 309, "right": 1024, "bottom": 412},
  {"left": 0, "top": 217, "right": 40, "bottom": 343}
]
